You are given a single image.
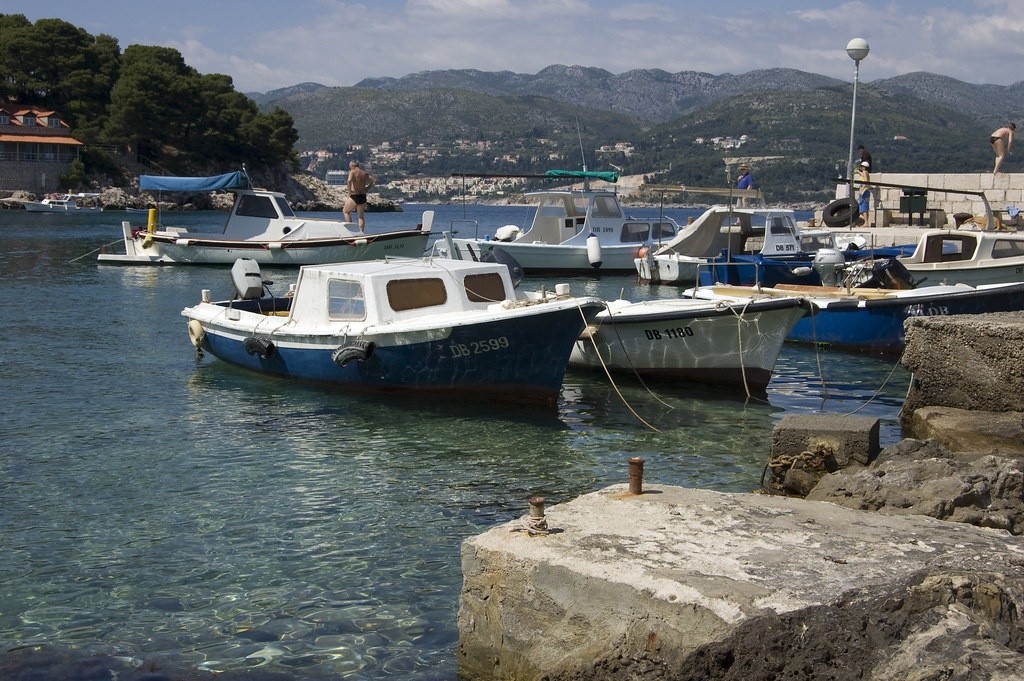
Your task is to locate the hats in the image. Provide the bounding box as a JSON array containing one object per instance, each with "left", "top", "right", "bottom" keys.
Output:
[
  {"left": 861, "top": 161, "right": 869, "bottom": 167},
  {"left": 737, "top": 164, "right": 751, "bottom": 170}
]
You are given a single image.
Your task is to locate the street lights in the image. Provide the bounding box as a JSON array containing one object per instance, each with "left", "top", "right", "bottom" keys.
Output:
[{"left": 845, "top": 38, "right": 870, "bottom": 198}]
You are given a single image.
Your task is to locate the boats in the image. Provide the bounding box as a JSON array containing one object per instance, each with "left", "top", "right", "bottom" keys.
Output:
[
  {"left": 0, "top": 190, "right": 38, "bottom": 210},
  {"left": 522, "top": 288, "right": 821, "bottom": 389},
  {"left": 434, "top": 188, "right": 682, "bottom": 275},
  {"left": 23, "top": 199, "right": 106, "bottom": 215},
  {"left": 180, "top": 253, "right": 607, "bottom": 405},
  {"left": 635, "top": 205, "right": 1024, "bottom": 353},
  {"left": 126, "top": 205, "right": 158, "bottom": 213},
  {"left": 97, "top": 186, "right": 435, "bottom": 266}
]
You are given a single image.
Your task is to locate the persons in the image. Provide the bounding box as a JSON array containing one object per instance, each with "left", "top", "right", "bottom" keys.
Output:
[
  {"left": 989, "top": 123, "right": 1016, "bottom": 174},
  {"left": 343, "top": 160, "right": 369, "bottom": 233},
  {"left": 639, "top": 243, "right": 650, "bottom": 259},
  {"left": 731, "top": 163, "right": 753, "bottom": 226},
  {"left": 853, "top": 145, "right": 872, "bottom": 227}
]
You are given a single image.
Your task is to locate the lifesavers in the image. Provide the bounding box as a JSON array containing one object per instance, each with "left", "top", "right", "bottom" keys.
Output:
[
  {"left": 821, "top": 196, "right": 861, "bottom": 229},
  {"left": 186, "top": 318, "right": 205, "bottom": 348}
]
